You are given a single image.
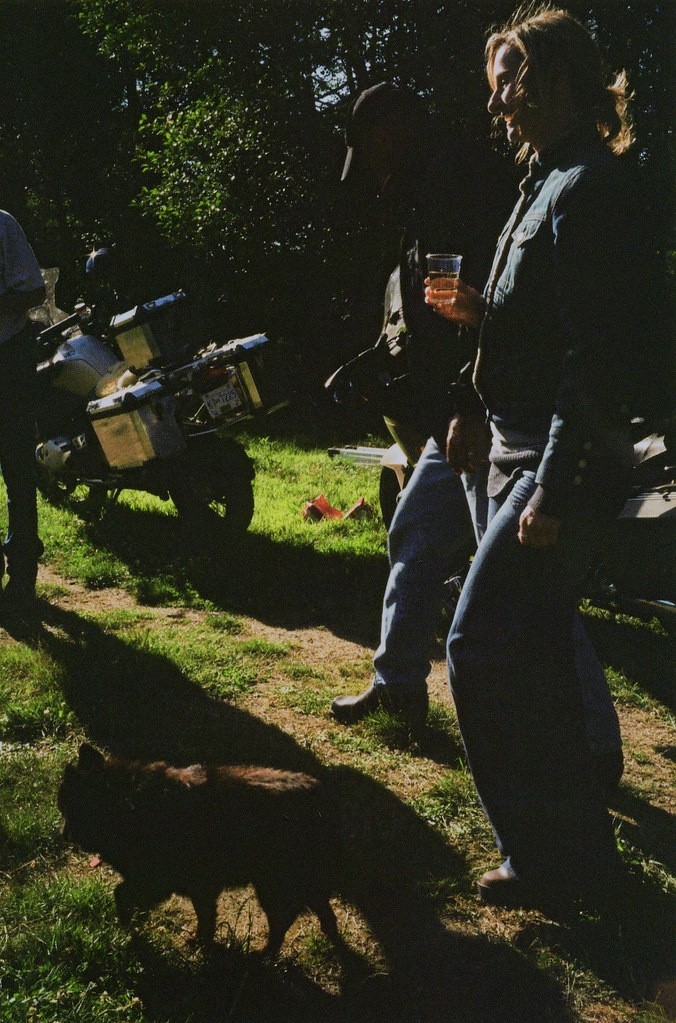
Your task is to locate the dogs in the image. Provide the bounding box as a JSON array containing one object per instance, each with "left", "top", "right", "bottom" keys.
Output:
[{"left": 54, "top": 743, "right": 376, "bottom": 962}]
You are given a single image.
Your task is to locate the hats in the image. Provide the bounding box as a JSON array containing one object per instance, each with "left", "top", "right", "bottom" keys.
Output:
[{"left": 338, "top": 80, "right": 420, "bottom": 180}]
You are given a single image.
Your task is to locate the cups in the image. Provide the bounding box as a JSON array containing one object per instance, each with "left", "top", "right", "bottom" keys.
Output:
[{"left": 425, "top": 254, "right": 462, "bottom": 306}]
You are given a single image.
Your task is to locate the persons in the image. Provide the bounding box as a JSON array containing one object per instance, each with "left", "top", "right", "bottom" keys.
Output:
[
  {"left": 322, "top": 80, "right": 502, "bottom": 720},
  {"left": 0, "top": 209, "right": 46, "bottom": 621},
  {"left": 423, "top": 10, "right": 642, "bottom": 904}
]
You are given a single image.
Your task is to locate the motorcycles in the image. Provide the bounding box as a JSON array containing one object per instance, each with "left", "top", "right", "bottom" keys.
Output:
[
  {"left": 322, "top": 206, "right": 676, "bottom": 635},
  {"left": 28, "top": 242, "right": 288, "bottom": 545}
]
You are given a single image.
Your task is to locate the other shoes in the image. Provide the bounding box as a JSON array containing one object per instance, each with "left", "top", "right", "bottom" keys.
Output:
[
  {"left": 332, "top": 684, "right": 427, "bottom": 723},
  {"left": 478, "top": 865, "right": 530, "bottom": 899},
  {"left": 6, "top": 565, "right": 39, "bottom": 576}
]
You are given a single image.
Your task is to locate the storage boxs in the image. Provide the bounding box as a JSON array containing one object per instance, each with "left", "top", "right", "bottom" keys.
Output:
[
  {"left": 107, "top": 288, "right": 211, "bottom": 375},
  {"left": 195, "top": 336, "right": 293, "bottom": 434},
  {"left": 88, "top": 381, "right": 188, "bottom": 471}
]
